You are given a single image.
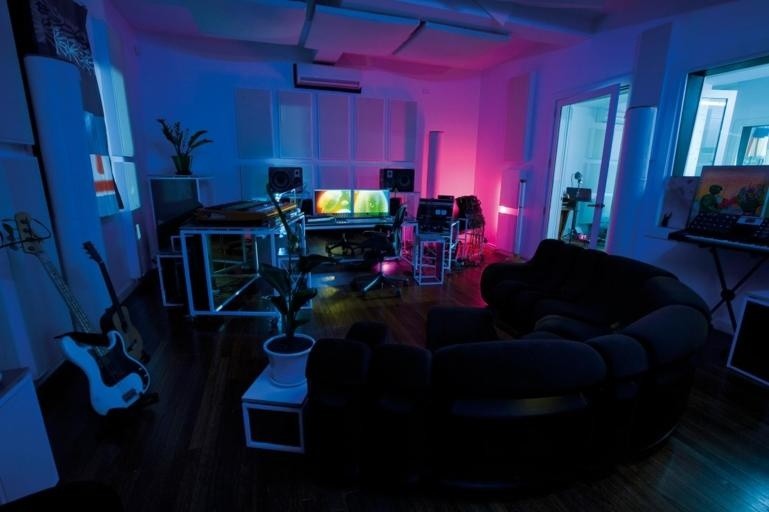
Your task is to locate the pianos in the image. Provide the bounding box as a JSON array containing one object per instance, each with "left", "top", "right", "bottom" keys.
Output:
[
  {"left": 195, "top": 200, "right": 297, "bottom": 221},
  {"left": 668, "top": 210, "right": 768, "bottom": 254}
]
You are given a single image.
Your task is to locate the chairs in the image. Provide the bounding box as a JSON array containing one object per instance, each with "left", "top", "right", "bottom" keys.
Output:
[{"left": 361, "top": 204, "right": 409, "bottom": 294}]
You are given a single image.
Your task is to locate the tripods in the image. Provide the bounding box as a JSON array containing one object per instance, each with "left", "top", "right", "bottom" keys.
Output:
[{"left": 562, "top": 201, "right": 580, "bottom": 244}]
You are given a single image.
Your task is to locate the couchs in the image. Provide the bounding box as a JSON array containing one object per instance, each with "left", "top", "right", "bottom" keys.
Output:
[{"left": 305, "top": 239, "right": 713, "bottom": 511}]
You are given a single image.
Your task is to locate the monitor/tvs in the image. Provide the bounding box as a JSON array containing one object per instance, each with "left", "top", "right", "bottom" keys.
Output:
[
  {"left": 353, "top": 188, "right": 390, "bottom": 218},
  {"left": 312, "top": 188, "right": 353, "bottom": 218}
]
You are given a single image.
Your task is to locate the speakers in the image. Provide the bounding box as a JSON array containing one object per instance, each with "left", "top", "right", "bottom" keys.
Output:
[
  {"left": 380, "top": 168, "right": 415, "bottom": 193},
  {"left": 268, "top": 166, "right": 303, "bottom": 194},
  {"left": 390, "top": 197, "right": 401, "bottom": 216},
  {"left": 301, "top": 199, "right": 313, "bottom": 216}
]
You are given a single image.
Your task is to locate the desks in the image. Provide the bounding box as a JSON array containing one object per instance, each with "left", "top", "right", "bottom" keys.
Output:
[
  {"left": 178, "top": 210, "right": 304, "bottom": 323},
  {"left": 305, "top": 216, "right": 402, "bottom": 263},
  {"left": 240, "top": 364, "right": 309, "bottom": 455},
  {"left": 669, "top": 232, "right": 769, "bottom": 333}
]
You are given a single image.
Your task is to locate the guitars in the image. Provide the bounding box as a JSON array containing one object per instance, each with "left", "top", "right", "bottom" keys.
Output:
[{"left": 15, "top": 212, "right": 151, "bottom": 417}]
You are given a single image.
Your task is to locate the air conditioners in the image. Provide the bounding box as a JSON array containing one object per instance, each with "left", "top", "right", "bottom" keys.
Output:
[{"left": 296, "top": 64, "right": 360, "bottom": 91}]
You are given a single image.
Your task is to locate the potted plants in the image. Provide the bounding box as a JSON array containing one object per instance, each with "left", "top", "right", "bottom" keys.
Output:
[
  {"left": 255, "top": 253, "right": 341, "bottom": 388},
  {"left": 156, "top": 117, "right": 212, "bottom": 175}
]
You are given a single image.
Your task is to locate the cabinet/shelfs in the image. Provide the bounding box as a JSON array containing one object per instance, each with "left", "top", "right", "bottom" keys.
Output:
[{"left": 405, "top": 217, "right": 468, "bottom": 287}]
